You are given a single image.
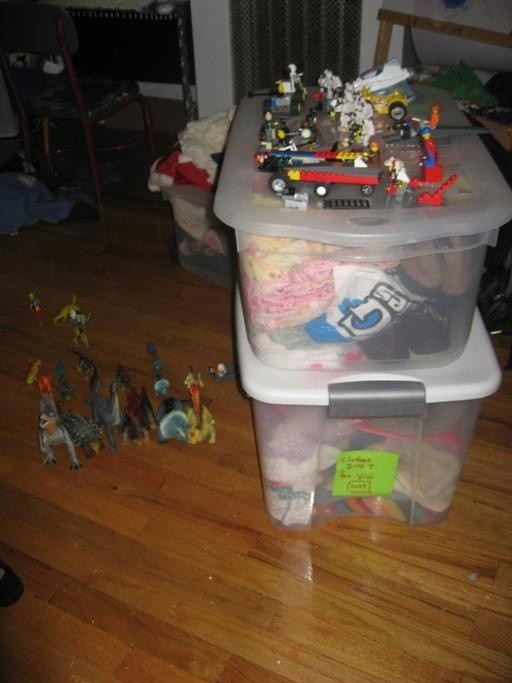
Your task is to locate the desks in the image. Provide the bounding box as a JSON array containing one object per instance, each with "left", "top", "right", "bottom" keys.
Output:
[{"left": 31, "top": 0, "right": 201, "bottom": 151}]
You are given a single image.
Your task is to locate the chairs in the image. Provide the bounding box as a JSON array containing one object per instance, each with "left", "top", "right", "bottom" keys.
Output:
[{"left": 1, "top": 0, "right": 157, "bottom": 220}]
